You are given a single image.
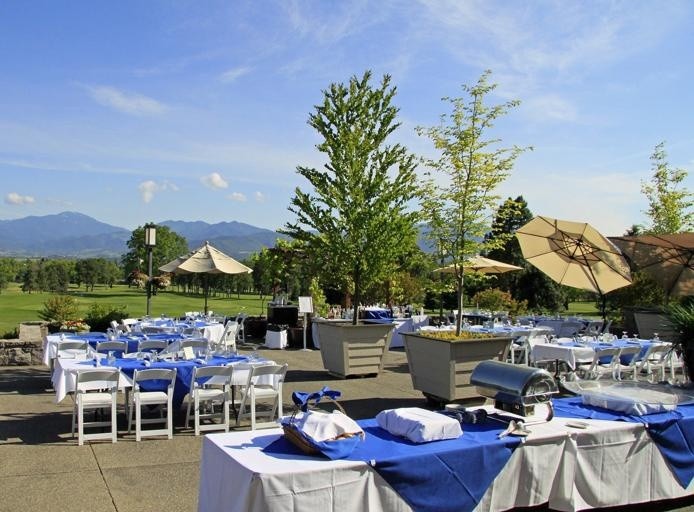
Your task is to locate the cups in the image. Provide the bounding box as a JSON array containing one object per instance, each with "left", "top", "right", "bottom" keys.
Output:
[{"left": 444, "top": 403, "right": 461, "bottom": 412}]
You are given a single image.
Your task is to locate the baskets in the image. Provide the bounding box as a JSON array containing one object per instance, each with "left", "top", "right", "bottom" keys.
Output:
[{"left": 282, "top": 393, "right": 364, "bottom": 456}]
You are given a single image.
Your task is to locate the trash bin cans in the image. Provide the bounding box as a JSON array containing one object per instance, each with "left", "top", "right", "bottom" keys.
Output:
[{"left": 288, "top": 327, "right": 304, "bottom": 350}]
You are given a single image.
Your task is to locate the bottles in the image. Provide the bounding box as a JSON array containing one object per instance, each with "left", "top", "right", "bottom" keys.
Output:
[
  {"left": 380, "top": 303, "right": 424, "bottom": 319},
  {"left": 274, "top": 293, "right": 289, "bottom": 305},
  {"left": 326, "top": 302, "right": 380, "bottom": 320},
  {"left": 73, "top": 351, "right": 78, "bottom": 359},
  {"left": 59, "top": 332, "right": 66, "bottom": 342}
]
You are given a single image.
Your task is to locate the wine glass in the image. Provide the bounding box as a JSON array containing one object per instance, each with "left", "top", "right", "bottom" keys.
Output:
[
  {"left": 547, "top": 330, "right": 660, "bottom": 347},
  {"left": 529, "top": 311, "right": 584, "bottom": 322},
  {"left": 434, "top": 317, "right": 536, "bottom": 333},
  {"left": 475, "top": 307, "right": 504, "bottom": 316},
  {"left": 86, "top": 312, "right": 261, "bottom": 367}
]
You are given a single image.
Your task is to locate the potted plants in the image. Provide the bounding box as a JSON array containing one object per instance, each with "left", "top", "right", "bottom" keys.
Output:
[
  {"left": 397, "top": 68, "right": 535, "bottom": 409},
  {"left": 268, "top": 68, "right": 429, "bottom": 379}
]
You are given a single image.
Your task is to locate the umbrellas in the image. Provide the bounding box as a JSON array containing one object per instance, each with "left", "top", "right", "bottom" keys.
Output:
[
  {"left": 430, "top": 254, "right": 525, "bottom": 311},
  {"left": 156, "top": 243, "right": 254, "bottom": 316},
  {"left": 605, "top": 231, "right": 694, "bottom": 306},
  {"left": 514, "top": 213, "right": 635, "bottom": 318}
]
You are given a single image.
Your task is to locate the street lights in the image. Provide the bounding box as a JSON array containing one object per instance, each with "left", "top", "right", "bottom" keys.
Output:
[{"left": 143, "top": 222, "right": 158, "bottom": 319}]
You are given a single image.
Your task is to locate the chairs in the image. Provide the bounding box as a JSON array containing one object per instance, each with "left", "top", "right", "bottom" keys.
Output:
[
  {"left": 453, "top": 309, "right": 459, "bottom": 321},
  {"left": 505, "top": 319, "right": 688, "bottom": 383},
  {"left": 50, "top": 312, "right": 288, "bottom": 447}
]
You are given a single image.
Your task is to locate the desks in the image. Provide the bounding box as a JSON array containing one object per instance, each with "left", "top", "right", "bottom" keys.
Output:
[
  {"left": 455, "top": 313, "right": 505, "bottom": 325},
  {"left": 196, "top": 385, "right": 694, "bottom": 512},
  {"left": 312, "top": 317, "right": 415, "bottom": 348},
  {"left": 411, "top": 314, "right": 429, "bottom": 333}
]
